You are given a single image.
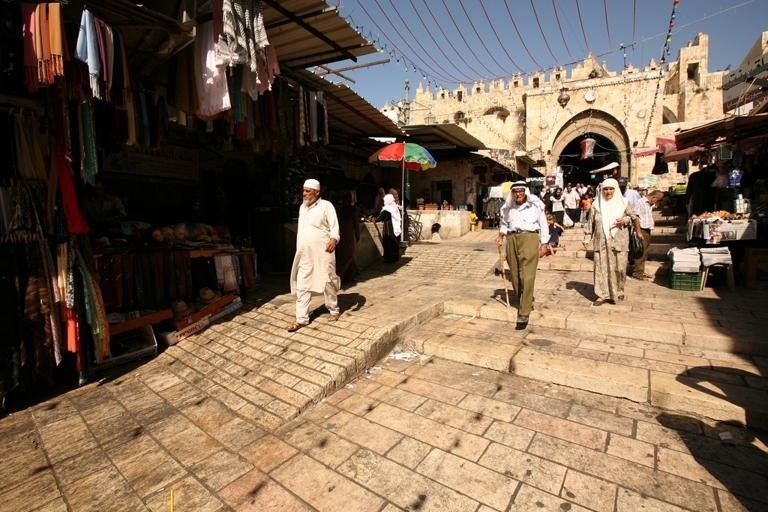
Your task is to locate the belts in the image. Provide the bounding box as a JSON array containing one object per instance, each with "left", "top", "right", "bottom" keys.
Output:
[{"left": 506, "top": 230, "right": 538, "bottom": 235}]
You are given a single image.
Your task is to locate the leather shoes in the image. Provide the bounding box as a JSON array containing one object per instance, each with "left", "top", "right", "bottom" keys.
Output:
[
  {"left": 514, "top": 321, "right": 529, "bottom": 330},
  {"left": 592, "top": 296, "right": 608, "bottom": 306}
]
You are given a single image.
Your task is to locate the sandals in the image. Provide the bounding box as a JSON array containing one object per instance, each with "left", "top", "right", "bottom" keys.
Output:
[
  {"left": 326, "top": 311, "right": 343, "bottom": 322},
  {"left": 287, "top": 319, "right": 311, "bottom": 332}
]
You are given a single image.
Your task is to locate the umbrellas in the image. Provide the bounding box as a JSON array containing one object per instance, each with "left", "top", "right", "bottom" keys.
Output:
[{"left": 367, "top": 140, "right": 438, "bottom": 241}]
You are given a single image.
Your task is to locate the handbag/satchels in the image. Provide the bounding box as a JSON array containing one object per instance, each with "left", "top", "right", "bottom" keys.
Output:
[{"left": 629, "top": 228, "right": 644, "bottom": 260}]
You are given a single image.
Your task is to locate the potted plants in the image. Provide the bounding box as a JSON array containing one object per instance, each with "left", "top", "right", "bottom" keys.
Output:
[{"left": 416, "top": 198, "right": 425, "bottom": 210}]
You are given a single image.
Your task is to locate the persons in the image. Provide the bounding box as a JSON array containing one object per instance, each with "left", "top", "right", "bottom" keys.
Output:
[
  {"left": 82, "top": 178, "right": 127, "bottom": 223},
  {"left": 169, "top": 196, "right": 202, "bottom": 223},
  {"left": 372, "top": 186, "right": 409, "bottom": 263},
  {"left": 465, "top": 188, "right": 476, "bottom": 210},
  {"left": 495, "top": 173, "right": 665, "bottom": 331},
  {"left": 286, "top": 178, "right": 341, "bottom": 331}
]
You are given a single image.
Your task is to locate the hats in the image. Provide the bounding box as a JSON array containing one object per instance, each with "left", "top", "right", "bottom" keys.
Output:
[
  {"left": 302, "top": 178, "right": 322, "bottom": 190},
  {"left": 170, "top": 298, "right": 194, "bottom": 318},
  {"left": 195, "top": 285, "right": 221, "bottom": 304}
]
